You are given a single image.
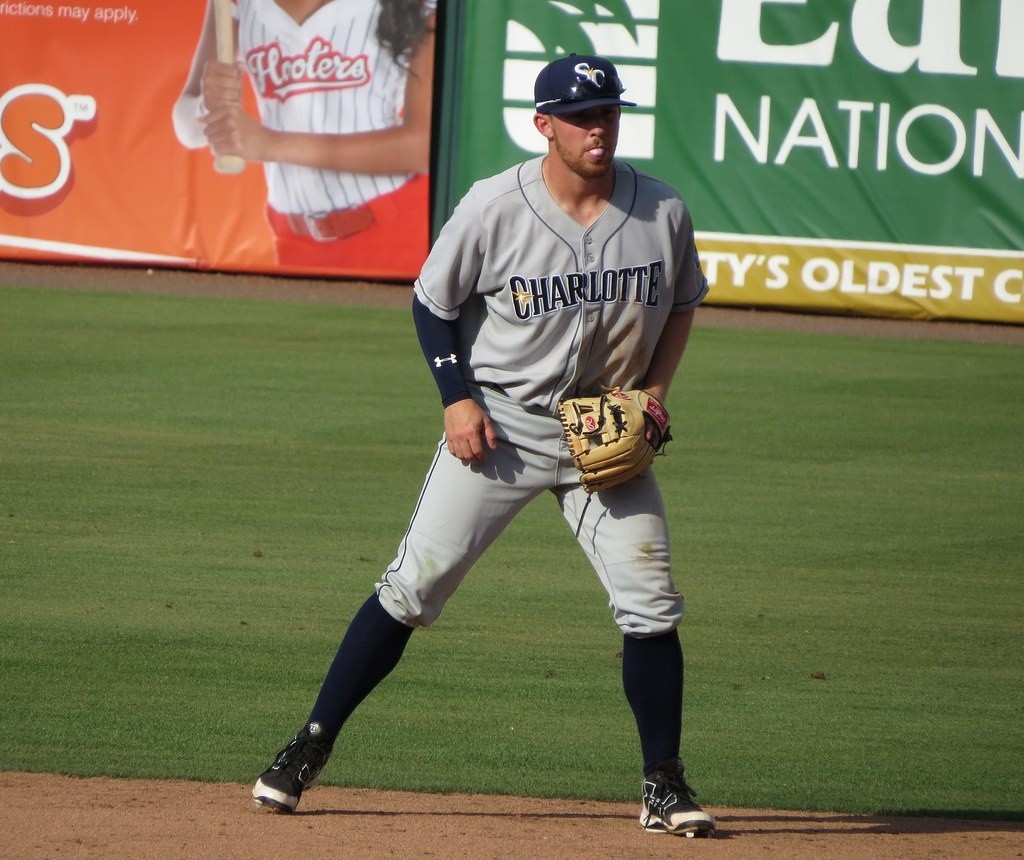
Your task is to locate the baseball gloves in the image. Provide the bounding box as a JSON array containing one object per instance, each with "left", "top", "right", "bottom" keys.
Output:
[{"left": 558, "top": 386, "right": 674, "bottom": 496}]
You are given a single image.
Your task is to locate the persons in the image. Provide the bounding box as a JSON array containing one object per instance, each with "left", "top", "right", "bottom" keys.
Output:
[
  {"left": 254, "top": 53, "right": 723, "bottom": 838},
  {"left": 172, "top": 0, "right": 436, "bottom": 277}
]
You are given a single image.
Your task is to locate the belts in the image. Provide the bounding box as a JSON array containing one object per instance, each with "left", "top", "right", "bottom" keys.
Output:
[{"left": 289, "top": 206, "right": 374, "bottom": 241}]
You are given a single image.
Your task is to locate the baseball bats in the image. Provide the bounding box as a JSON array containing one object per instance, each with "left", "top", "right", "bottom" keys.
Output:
[{"left": 206, "top": 1, "right": 247, "bottom": 176}]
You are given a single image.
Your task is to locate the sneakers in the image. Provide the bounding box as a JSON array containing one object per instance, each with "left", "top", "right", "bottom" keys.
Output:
[
  {"left": 641, "top": 766, "right": 716, "bottom": 835},
  {"left": 256, "top": 726, "right": 334, "bottom": 813}
]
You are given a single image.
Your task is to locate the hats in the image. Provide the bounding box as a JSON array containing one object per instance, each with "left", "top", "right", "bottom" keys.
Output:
[{"left": 535, "top": 54, "right": 635, "bottom": 115}]
